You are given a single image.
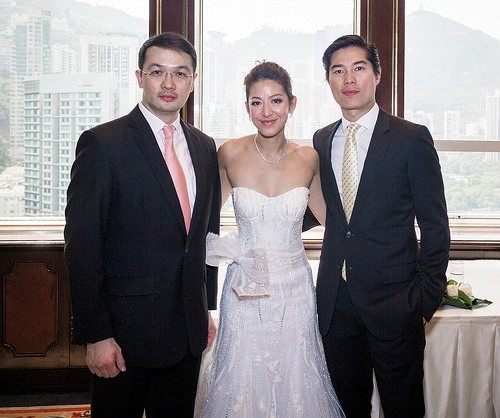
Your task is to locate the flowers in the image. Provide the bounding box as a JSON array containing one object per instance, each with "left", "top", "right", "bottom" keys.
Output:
[{"left": 438, "top": 280, "right": 493, "bottom": 310}]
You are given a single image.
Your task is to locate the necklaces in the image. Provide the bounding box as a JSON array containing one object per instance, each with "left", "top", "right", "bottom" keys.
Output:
[{"left": 253, "top": 133, "right": 288, "bottom": 165}]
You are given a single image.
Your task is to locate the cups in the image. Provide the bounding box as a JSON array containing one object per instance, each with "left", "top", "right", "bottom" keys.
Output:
[{"left": 453, "top": 261, "right": 466, "bottom": 284}]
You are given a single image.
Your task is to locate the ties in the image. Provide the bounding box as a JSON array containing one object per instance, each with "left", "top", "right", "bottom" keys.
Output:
[
  {"left": 341, "top": 124, "right": 358, "bottom": 281},
  {"left": 162, "top": 125, "right": 191, "bottom": 235}
]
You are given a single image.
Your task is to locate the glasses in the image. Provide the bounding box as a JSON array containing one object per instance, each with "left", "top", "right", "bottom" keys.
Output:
[{"left": 138, "top": 68, "right": 194, "bottom": 80}]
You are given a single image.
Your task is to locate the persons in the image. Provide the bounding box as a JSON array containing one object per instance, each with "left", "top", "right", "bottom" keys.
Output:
[
  {"left": 191, "top": 61, "right": 348, "bottom": 418},
  {"left": 302, "top": 35, "right": 451, "bottom": 415},
  {"left": 62, "top": 30, "right": 223, "bottom": 418}
]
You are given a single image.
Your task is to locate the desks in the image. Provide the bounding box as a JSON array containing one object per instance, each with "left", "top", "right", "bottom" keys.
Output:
[{"left": 307, "top": 259, "right": 500, "bottom": 417}]
